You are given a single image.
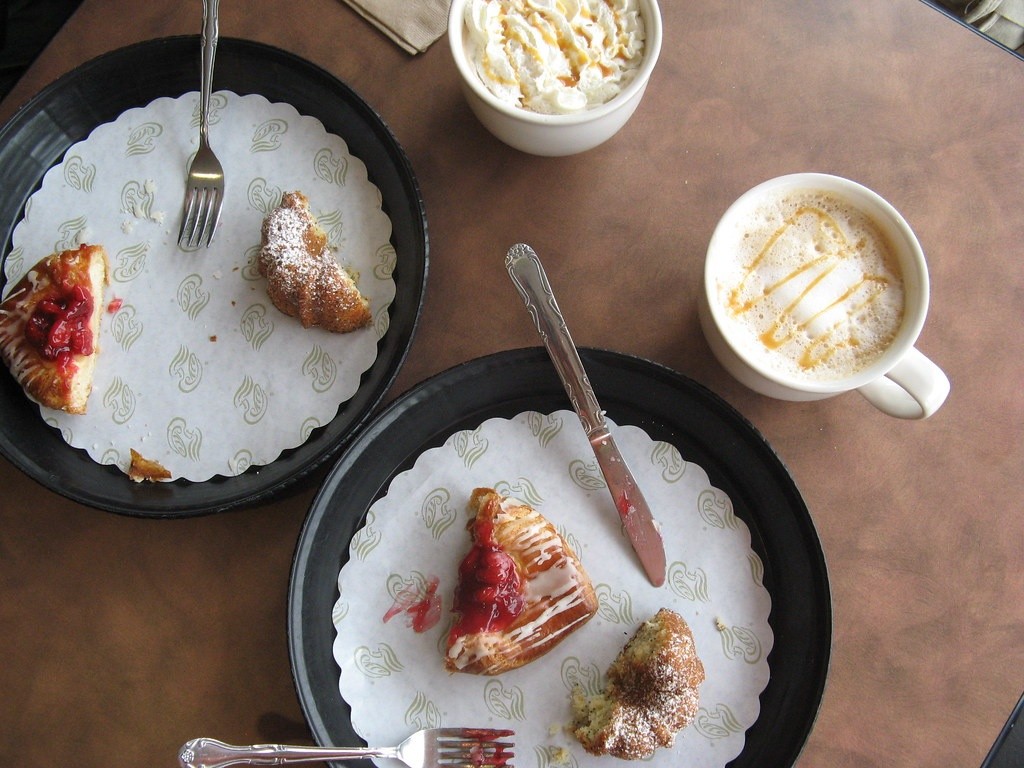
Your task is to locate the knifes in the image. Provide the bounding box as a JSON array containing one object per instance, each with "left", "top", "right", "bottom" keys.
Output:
[{"left": 505, "top": 243, "right": 667, "bottom": 588}]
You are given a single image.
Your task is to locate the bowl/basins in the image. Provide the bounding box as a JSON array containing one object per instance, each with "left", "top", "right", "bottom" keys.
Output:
[{"left": 447, "top": 0, "right": 662, "bottom": 156}]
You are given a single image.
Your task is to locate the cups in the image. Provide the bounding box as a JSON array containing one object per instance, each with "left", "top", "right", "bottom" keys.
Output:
[{"left": 699, "top": 173, "right": 950, "bottom": 420}]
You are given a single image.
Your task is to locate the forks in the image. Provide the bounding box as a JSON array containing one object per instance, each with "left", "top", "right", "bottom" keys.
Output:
[
  {"left": 176, "top": 728, "right": 515, "bottom": 768},
  {"left": 177, "top": 1, "right": 225, "bottom": 248}
]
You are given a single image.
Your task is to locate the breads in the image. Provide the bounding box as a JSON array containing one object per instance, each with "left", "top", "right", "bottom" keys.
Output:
[
  {"left": 259, "top": 190, "right": 372, "bottom": 333},
  {"left": 573, "top": 608, "right": 706, "bottom": 762}
]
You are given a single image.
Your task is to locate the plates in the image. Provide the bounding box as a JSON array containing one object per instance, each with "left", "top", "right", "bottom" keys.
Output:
[
  {"left": 287, "top": 345, "right": 832, "bottom": 768},
  {"left": 2, "top": 36, "right": 429, "bottom": 518}
]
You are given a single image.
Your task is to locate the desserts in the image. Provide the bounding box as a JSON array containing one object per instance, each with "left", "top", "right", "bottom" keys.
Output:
[
  {"left": 0, "top": 245, "right": 109, "bottom": 414},
  {"left": 448, "top": 0, "right": 662, "bottom": 158},
  {"left": 442, "top": 488, "right": 598, "bottom": 676}
]
